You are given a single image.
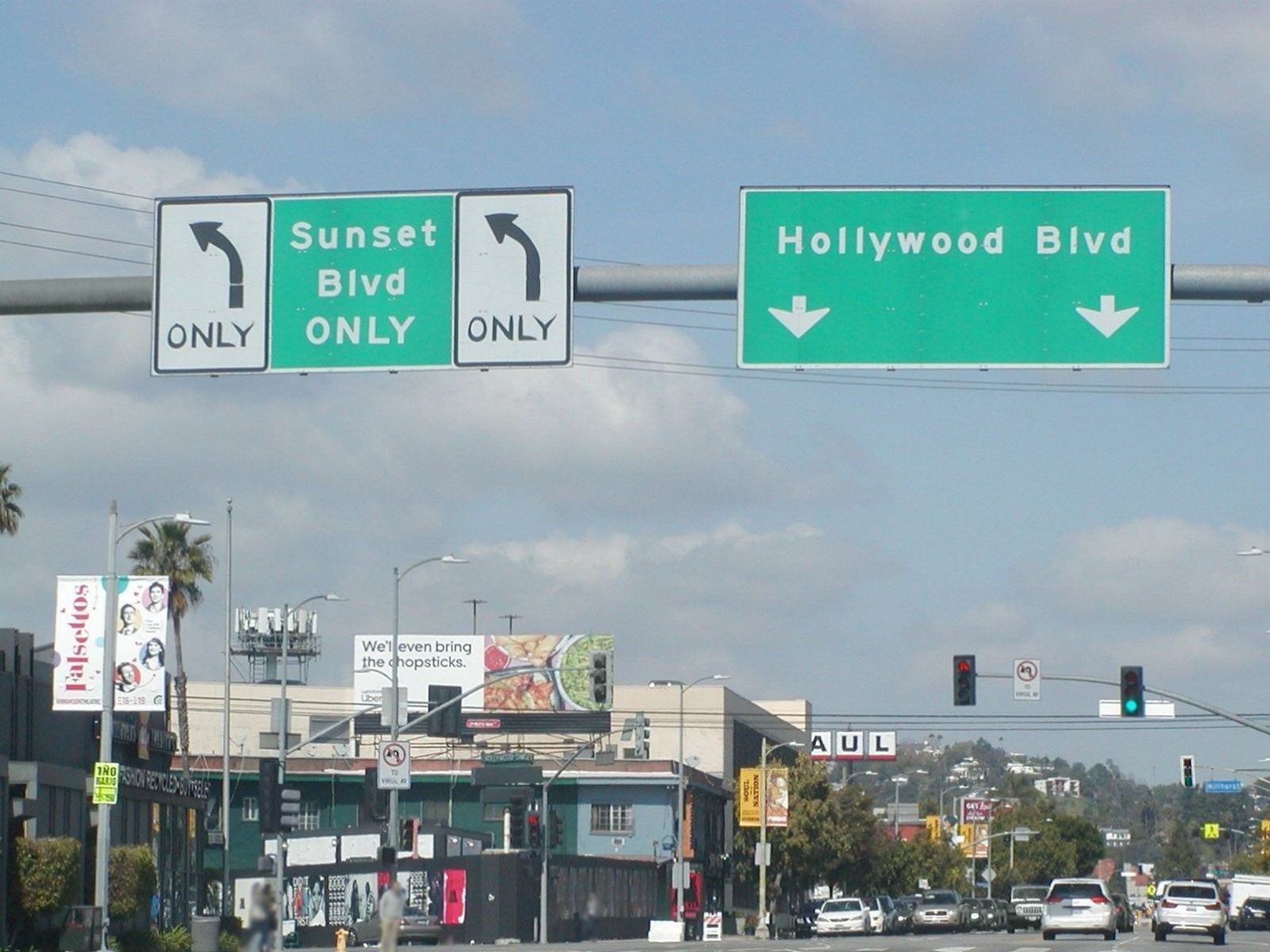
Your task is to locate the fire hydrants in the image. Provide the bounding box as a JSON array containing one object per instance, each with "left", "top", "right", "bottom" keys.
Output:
[{"left": 334, "top": 928, "right": 349, "bottom": 952}]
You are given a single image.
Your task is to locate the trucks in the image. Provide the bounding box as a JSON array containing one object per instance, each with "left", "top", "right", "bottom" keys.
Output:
[{"left": 1227, "top": 875, "right": 1270, "bottom": 930}]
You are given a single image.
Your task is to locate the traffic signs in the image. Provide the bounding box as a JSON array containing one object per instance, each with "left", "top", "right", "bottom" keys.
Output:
[
  {"left": 154, "top": 186, "right": 574, "bottom": 373},
  {"left": 737, "top": 181, "right": 1175, "bottom": 371}
]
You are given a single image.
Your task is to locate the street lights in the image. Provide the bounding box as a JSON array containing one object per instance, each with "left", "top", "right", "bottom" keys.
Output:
[
  {"left": 755, "top": 737, "right": 804, "bottom": 941},
  {"left": 953, "top": 785, "right": 999, "bottom": 823},
  {"left": 939, "top": 783, "right": 970, "bottom": 826},
  {"left": 352, "top": 554, "right": 469, "bottom": 948},
  {"left": 1010, "top": 819, "right": 1052, "bottom": 872},
  {"left": 676, "top": 673, "right": 732, "bottom": 921},
  {"left": 91, "top": 499, "right": 213, "bottom": 948},
  {"left": 272, "top": 592, "right": 349, "bottom": 952},
  {"left": 894, "top": 770, "right": 929, "bottom": 841}
]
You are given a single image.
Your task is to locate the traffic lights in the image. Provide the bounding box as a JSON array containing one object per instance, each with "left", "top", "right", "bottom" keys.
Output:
[
  {"left": 504, "top": 796, "right": 563, "bottom": 851},
  {"left": 953, "top": 655, "right": 974, "bottom": 708},
  {"left": 635, "top": 711, "right": 652, "bottom": 757},
  {"left": 1181, "top": 755, "right": 1195, "bottom": 789},
  {"left": 1120, "top": 665, "right": 1146, "bottom": 719},
  {"left": 1214, "top": 865, "right": 1220, "bottom": 877}
]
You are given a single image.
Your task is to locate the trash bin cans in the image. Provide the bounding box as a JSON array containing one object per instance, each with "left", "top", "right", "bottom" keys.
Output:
[
  {"left": 190, "top": 915, "right": 222, "bottom": 952},
  {"left": 57, "top": 905, "right": 102, "bottom": 951}
]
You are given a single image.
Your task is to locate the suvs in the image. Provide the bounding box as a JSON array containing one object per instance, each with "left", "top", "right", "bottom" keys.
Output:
[{"left": 1006, "top": 885, "right": 1049, "bottom": 934}]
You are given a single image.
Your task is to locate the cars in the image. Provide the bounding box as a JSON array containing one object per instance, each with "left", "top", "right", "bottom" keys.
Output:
[
  {"left": 914, "top": 888, "right": 966, "bottom": 933},
  {"left": 962, "top": 898, "right": 1009, "bottom": 933},
  {"left": 346, "top": 902, "right": 442, "bottom": 948},
  {"left": 814, "top": 898, "right": 872, "bottom": 938},
  {"left": 865, "top": 894, "right": 919, "bottom": 935},
  {"left": 1040, "top": 877, "right": 1137, "bottom": 943},
  {"left": 794, "top": 900, "right": 824, "bottom": 939},
  {"left": 1146, "top": 879, "right": 1227, "bottom": 945},
  {"left": 1236, "top": 896, "right": 1270, "bottom": 927}
]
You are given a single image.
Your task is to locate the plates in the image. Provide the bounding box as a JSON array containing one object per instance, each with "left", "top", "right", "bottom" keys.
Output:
[
  {"left": 532, "top": 671, "right": 558, "bottom": 711},
  {"left": 484, "top": 645, "right": 510, "bottom": 671}
]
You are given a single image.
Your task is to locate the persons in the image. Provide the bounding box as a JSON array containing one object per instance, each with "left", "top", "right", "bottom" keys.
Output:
[
  {"left": 118, "top": 604, "right": 138, "bottom": 635},
  {"left": 376, "top": 881, "right": 403, "bottom": 952},
  {"left": 117, "top": 662, "right": 139, "bottom": 692},
  {"left": 143, "top": 638, "right": 165, "bottom": 671},
  {"left": 365, "top": 881, "right": 375, "bottom": 921},
  {"left": 351, "top": 880, "right": 360, "bottom": 923},
  {"left": 146, "top": 582, "right": 166, "bottom": 612},
  {"left": 309, "top": 877, "right": 328, "bottom": 926}
]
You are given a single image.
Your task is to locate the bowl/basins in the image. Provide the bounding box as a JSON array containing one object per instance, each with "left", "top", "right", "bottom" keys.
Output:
[
  {"left": 554, "top": 635, "right": 614, "bottom": 711},
  {"left": 490, "top": 635, "right": 570, "bottom": 661}
]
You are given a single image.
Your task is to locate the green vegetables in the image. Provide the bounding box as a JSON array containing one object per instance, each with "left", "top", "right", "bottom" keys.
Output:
[{"left": 561, "top": 635, "right": 614, "bottom": 710}]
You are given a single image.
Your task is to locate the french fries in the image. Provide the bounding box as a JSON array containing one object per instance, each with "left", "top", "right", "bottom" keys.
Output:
[{"left": 490, "top": 635, "right": 563, "bottom": 667}]
